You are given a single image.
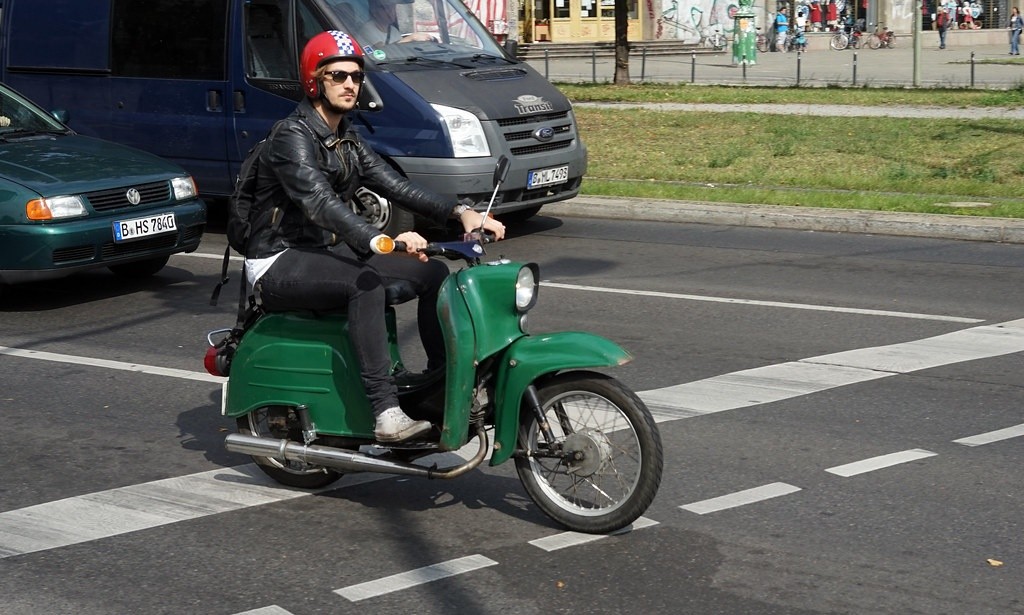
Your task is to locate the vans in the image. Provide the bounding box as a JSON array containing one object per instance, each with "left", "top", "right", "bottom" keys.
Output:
[{"left": 0, "top": 0, "right": 588, "bottom": 239}]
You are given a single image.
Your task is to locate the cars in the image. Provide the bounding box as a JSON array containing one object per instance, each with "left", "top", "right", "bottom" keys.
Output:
[{"left": 0, "top": 82, "right": 207, "bottom": 283}]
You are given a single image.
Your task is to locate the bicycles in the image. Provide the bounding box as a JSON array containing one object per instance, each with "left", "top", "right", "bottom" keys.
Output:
[
  {"left": 830, "top": 16, "right": 895, "bottom": 50},
  {"left": 698, "top": 30, "right": 727, "bottom": 50},
  {"left": 756, "top": 27, "right": 771, "bottom": 53}
]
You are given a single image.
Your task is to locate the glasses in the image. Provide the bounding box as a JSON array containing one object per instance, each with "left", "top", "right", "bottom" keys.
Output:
[{"left": 321, "top": 70, "right": 364, "bottom": 85}]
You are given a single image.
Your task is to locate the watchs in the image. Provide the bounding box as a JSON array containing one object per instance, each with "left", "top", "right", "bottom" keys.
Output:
[{"left": 456, "top": 202, "right": 473, "bottom": 218}]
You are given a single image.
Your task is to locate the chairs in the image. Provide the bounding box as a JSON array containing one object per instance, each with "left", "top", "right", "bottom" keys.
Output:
[{"left": 246, "top": 29, "right": 294, "bottom": 80}]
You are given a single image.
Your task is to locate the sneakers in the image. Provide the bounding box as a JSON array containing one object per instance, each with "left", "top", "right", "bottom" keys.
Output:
[{"left": 375, "top": 406, "right": 431, "bottom": 443}]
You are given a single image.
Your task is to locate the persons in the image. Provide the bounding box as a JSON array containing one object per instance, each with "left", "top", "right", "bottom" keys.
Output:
[
  {"left": 836, "top": 20, "right": 845, "bottom": 35},
  {"left": 1007, "top": 6, "right": 1024, "bottom": 55},
  {"left": 963, "top": 1, "right": 978, "bottom": 30},
  {"left": 794, "top": 12, "right": 808, "bottom": 53},
  {"left": 356, "top": 0, "right": 436, "bottom": 49},
  {"left": 776, "top": 6, "right": 789, "bottom": 55},
  {"left": 946, "top": 0, "right": 963, "bottom": 28},
  {"left": 937, "top": 6, "right": 951, "bottom": 49},
  {"left": 0, "top": 116, "right": 11, "bottom": 127},
  {"left": 806, "top": 0, "right": 838, "bottom": 31},
  {"left": 262, "top": 29, "right": 508, "bottom": 443}
]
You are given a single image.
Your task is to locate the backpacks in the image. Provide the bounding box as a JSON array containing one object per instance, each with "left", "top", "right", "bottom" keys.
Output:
[
  {"left": 773, "top": 19, "right": 781, "bottom": 29},
  {"left": 226, "top": 116, "right": 359, "bottom": 259}
]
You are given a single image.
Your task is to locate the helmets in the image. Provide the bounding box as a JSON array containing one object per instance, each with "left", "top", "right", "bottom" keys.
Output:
[{"left": 298, "top": 31, "right": 366, "bottom": 102}]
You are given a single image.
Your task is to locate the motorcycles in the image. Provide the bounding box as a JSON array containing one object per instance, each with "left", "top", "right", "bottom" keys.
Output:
[{"left": 204, "top": 154, "right": 663, "bottom": 534}]
